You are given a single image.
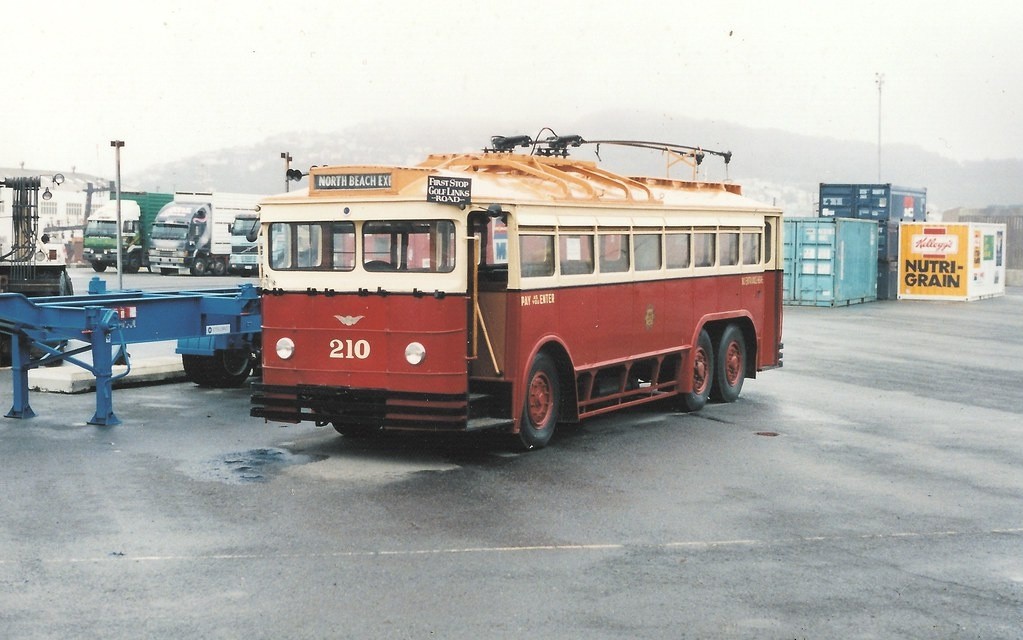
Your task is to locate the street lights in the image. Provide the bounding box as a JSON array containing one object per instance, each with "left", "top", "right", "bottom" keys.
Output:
[
  {"left": 280, "top": 151, "right": 292, "bottom": 192},
  {"left": 110, "top": 139, "right": 125, "bottom": 290},
  {"left": 875, "top": 67, "right": 899, "bottom": 179}
]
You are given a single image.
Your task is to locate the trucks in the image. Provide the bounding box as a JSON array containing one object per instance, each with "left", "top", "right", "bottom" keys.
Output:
[
  {"left": 227, "top": 213, "right": 287, "bottom": 278},
  {"left": 82, "top": 190, "right": 174, "bottom": 273},
  {"left": 147, "top": 190, "right": 273, "bottom": 277},
  {"left": 341, "top": 228, "right": 623, "bottom": 272}
]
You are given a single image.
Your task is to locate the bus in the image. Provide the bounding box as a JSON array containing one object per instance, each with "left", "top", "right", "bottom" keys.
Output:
[{"left": 251, "top": 134, "right": 786, "bottom": 449}]
